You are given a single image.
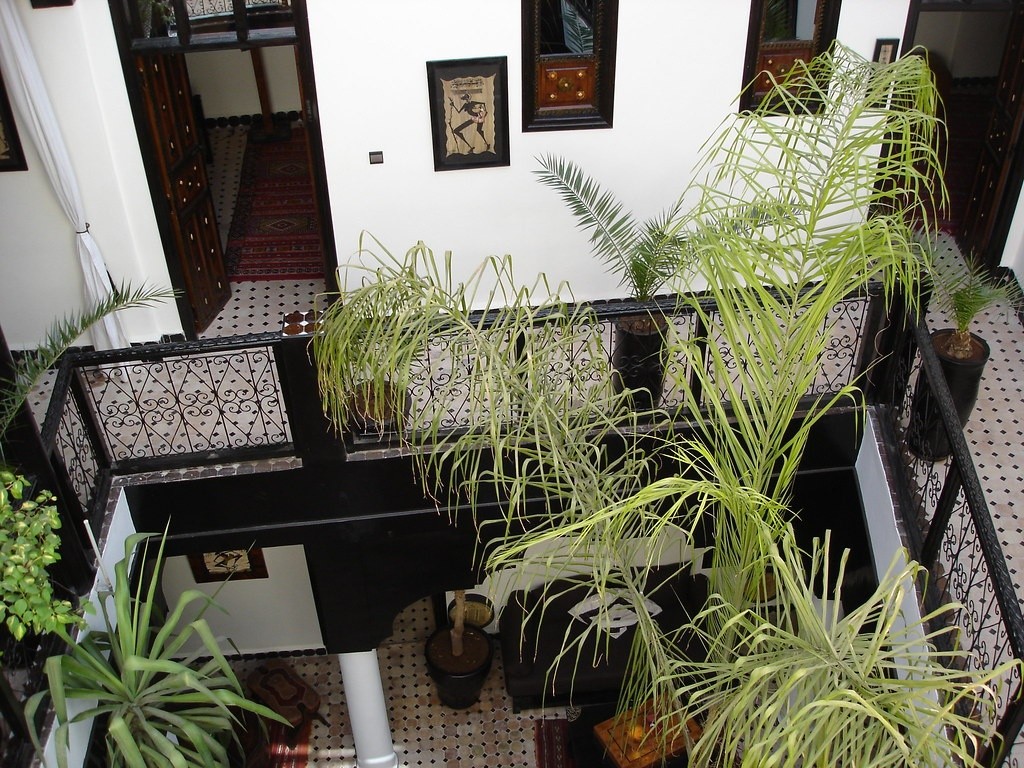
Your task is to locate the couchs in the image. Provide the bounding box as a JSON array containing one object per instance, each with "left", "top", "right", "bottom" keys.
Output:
[{"left": 499, "top": 560, "right": 741, "bottom": 713}]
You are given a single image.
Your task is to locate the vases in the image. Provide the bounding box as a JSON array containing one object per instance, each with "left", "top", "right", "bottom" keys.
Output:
[
  {"left": 344, "top": 379, "right": 412, "bottom": 436},
  {"left": 425, "top": 624, "right": 494, "bottom": 710}
]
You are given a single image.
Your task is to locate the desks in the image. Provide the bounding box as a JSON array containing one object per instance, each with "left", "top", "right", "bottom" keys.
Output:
[{"left": 247, "top": 658, "right": 331, "bottom": 750}]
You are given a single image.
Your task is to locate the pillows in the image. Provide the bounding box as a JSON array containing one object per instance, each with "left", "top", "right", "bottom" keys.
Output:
[{"left": 568, "top": 588, "right": 662, "bottom": 639}]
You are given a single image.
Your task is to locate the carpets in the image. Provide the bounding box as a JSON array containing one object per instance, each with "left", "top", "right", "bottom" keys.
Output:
[
  {"left": 535, "top": 719, "right": 575, "bottom": 768},
  {"left": 266, "top": 718, "right": 311, "bottom": 768},
  {"left": 224, "top": 127, "right": 326, "bottom": 281}
]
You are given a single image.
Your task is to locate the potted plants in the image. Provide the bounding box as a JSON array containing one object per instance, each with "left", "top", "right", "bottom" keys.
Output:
[
  {"left": 531, "top": 152, "right": 807, "bottom": 414},
  {"left": 907, "top": 250, "right": 1024, "bottom": 461},
  {"left": 23, "top": 509, "right": 296, "bottom": 768}
]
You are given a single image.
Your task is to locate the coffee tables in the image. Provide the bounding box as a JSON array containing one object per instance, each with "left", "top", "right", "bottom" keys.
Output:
[{"left": 566, "top": 693, "right": 718, "bottom": 768}]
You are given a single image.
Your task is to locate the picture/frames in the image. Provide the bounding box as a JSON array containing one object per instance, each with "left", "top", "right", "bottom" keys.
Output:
[
  {"left": 426, "top": 56, "right": 510, "bottom": 172},
  {"left": 0, "top": 68, "right": 30, "bottom": 172},
  {"left": 186, "top": 547, "right": 269, "bottom": 584}
]
